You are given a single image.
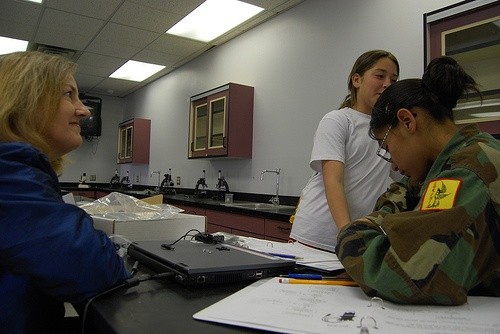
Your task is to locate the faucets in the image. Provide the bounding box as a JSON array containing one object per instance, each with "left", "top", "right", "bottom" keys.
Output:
[
  {"left": 260, "top": 168, "right": 280, "bottom": 207},
  {"left": 150, "top": 171, "right": 160, "bottom": 192}
]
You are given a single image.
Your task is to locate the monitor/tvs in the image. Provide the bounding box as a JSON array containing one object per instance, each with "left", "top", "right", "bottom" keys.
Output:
[{"left": 78, "top": 101, "right": 102, "bottom": 136}]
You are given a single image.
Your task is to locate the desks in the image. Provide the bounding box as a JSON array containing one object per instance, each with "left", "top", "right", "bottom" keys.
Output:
[{"left": 93, "top": 256, "right": 273, "bottom": 334}]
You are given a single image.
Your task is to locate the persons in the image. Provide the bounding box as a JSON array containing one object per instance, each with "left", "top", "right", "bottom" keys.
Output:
[
  {"left": 288, "top": 50, "right": 400, "bottom": 254},
  {"left": 337, "top": 56, "right": 500, "bottom": 305},
  {"left": 0, "top": 51, "right": 131, "bottom": 327}
]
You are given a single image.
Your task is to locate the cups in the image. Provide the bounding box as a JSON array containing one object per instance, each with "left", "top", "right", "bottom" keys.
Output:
[{"left": 225, "top": 194, "right": 233, "bottom": 205}]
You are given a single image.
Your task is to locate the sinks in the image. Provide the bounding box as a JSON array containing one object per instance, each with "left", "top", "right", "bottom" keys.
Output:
[
  {"left": 220, "top": 202, "right": 298, "bottom": 211},
  {"left": 124, "top": 190, "right": 147, "bottom": 194}
]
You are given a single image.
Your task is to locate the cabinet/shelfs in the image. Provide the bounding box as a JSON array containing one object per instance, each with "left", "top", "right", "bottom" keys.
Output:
[
  {"left": 174, "top": 204, "right": 297, "bottom": 243},
  {"left": 188, "top": 82, "right": 254, "bottom": 160},
  {"left": 117, "top": 119, "right": 151, "bottom": 165},
  {"left": 423, "top": 0, "right": 500, "bottom": 134}
]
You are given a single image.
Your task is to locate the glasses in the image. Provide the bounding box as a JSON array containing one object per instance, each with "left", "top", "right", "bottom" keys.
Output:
[{"left": 375, "top": 112, "right": 419, "bottom": 163}]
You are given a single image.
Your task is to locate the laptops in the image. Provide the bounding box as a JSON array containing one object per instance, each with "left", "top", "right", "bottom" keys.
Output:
[{"left": 127, "top": 239, "right": 296, "bottom": 290}]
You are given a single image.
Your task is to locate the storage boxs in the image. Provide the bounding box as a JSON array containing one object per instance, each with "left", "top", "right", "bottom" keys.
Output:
[{"left": 91, "top": 213, "right": 208, "bottom": 243}]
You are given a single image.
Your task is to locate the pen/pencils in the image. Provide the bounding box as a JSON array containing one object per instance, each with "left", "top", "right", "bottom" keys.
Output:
[
  {"left": 280, "top": 274, "right": 337, "bottom": 279},
  {"left": 269, "top": 252, "right": 307, "bottom": 259},
  {"left": 279, "top": 279, "right": 358, "bottom": 285}
]
